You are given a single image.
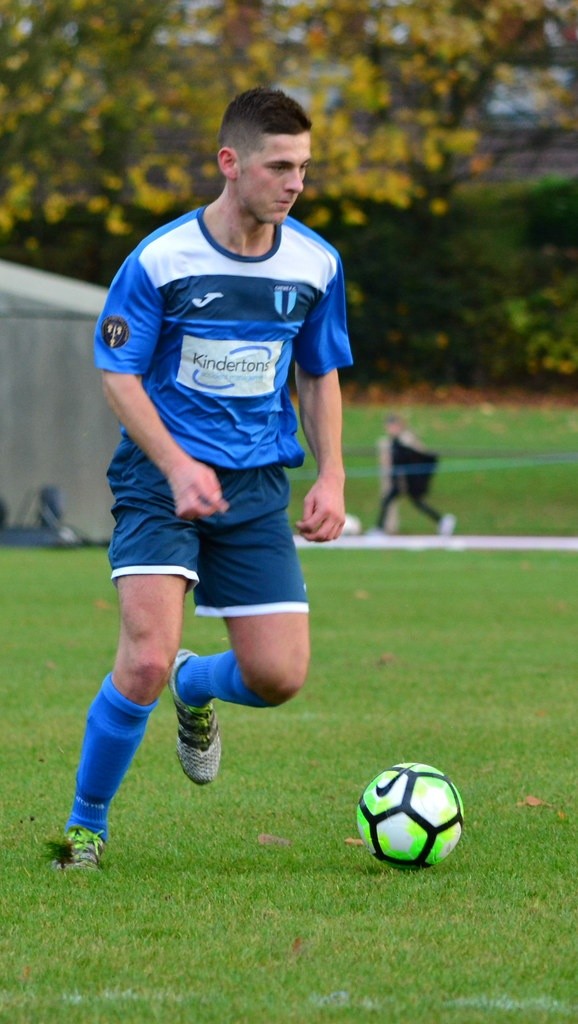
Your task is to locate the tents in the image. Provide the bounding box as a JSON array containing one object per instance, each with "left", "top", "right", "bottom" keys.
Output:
[{"left": 0, "top": 260, "right": 129, "bottom": 543}]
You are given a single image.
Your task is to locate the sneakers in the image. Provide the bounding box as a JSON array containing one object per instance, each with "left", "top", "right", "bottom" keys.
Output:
[
  {"left": 49, "top": 827, "right": 107, "bottom": 874},
  {"left": 165, "top": 647, "right": 222, "bottom": 785}
]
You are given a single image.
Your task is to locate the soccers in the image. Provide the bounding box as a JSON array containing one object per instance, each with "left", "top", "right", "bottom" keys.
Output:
[{"left": 357, "top": 764, "right": 465, "bottom": 874}]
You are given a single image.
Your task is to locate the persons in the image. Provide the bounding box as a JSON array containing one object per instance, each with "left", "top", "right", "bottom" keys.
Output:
[
  {"left": 367, "top": 415, "right": 458, "bottom": 536},
  {"left": 54, "top": 88, "right": 352, "bottom": 877}
]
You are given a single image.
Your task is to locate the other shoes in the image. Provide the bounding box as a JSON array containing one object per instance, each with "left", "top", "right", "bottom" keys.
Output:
[
  {"left": 436, "top": 513, "right": 457, "bottom": 535},
  {"left": 364, "top": 525, "right": 387, "bottom": 538}
]
